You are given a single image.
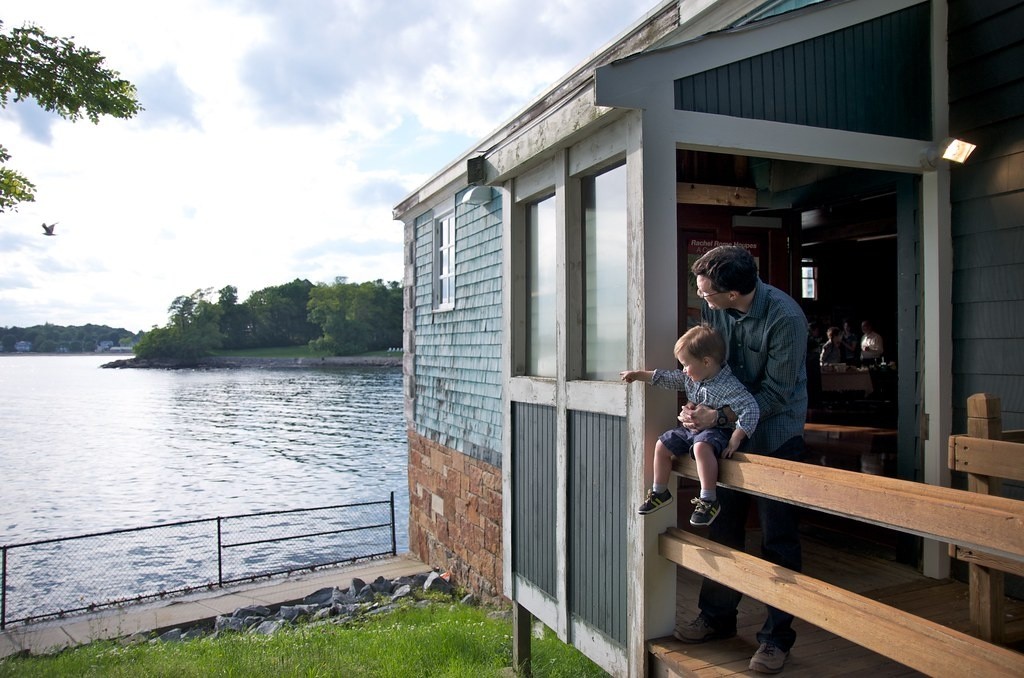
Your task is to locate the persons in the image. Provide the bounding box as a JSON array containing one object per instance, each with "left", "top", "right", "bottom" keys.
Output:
[
  {"left": 808, "top": 321, "right": 819, "bottom": 356},
  {"left": 820, "top": 327, "right": 847, "bottom": 365},
  {"left": 841, "top": 322, "right": 856, "bottom": 363},
  {"left": 619, "top": 327, "right": 759, "bottom": 525},
  {"left": 861, "top": 321, "right": 883, "bottom": 364},
  {"left": 673, "top": 245, "right": 807, "bottom": 672}
]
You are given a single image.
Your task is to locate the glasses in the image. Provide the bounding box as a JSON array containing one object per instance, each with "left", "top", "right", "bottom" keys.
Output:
[{"left": 697, "top": 289, "right": 719, "bottom": 299}]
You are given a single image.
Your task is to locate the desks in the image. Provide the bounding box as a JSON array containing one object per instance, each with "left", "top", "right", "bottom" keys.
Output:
[{"left": 821, "top": 361, "right": 896, "bottom": 411}]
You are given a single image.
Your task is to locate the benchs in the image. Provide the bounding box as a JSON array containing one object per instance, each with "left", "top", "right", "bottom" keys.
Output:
[{"left": 804, "top": 422, "right": 898, "bottom": 477}]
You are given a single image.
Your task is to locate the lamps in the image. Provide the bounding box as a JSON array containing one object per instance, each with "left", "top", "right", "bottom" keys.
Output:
[
  {"left": 461, "top": 186, "right": 493, "bottom": 206},
  {"left": 919, "top": 137, "right": 978, "bottom": 170}
]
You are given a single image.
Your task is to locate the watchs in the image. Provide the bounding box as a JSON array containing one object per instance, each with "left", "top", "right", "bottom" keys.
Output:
[{"left": 716, "top": 408, "right": 728, "bottom": 427}]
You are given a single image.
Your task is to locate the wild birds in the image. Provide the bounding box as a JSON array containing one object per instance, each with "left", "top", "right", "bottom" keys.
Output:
[{"left": 42, "top": 222, "right": 60, "bottom": 236}]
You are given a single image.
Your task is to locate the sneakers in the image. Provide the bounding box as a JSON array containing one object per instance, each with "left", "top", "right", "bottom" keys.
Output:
[
  {"left": 673, "top": 616, "right": 738, "bottom": 644},
  {"left": 690, "top": 497, "right": 720, "bottom": 528},
  {"left": 749, "top": 643, "right": 792, "bottom": 674},
  {"left": 638, "top": 489, "right": 672, "bottom": 514}
]
